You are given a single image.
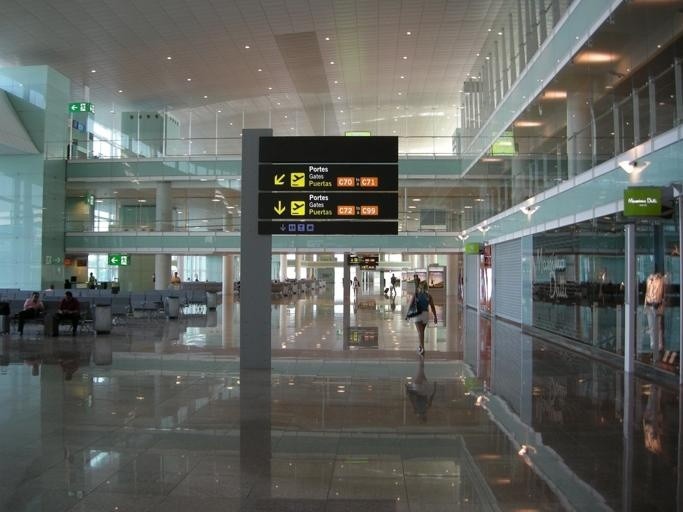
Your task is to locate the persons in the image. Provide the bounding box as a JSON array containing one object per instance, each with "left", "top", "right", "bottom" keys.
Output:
[
  {"left": 641, "top": 271, "right": 665, "bottom": 365},
  {"left": 388, "top": 292, "right": 397, "bottom": 312},
  {"left": 50, "top": 290, "right": 82, "bottom": 338},
  {"left": 414, "top": 274, "right": 420, "bottom": 287},
  {"left": 643, "top": 383, "right": 665, "bottom": 454},
  {"left": 404, "top": 355, "right": 436, "bottom": 427},
  {"left": 17, "top": 337, "right": 46, "bottom": 376},
  {"left": 352, "top": 276, "right": 360, "bottom": 298},
  {"left": 52, "top": 340, "right": 82, "bottom": 381},
  {"left": 170, "top": 271, "right": 181, "bottom": 290},
  {"left": 353, "top": 298, "right": 359, "bottom": 314},
  {"left": 14, "top": 291, "right": 45, "bottom": 335},
  {"left": 88, "top": 272, "right": 97, "bottom": 290},
  {"left": 389, "top": 273, "right": 396, "bottom": 292},
  {"left": 403, "top": 280, "right": 437, "bottom": 356},
  {"left": 63, "top": 279, "right": 71, "bottom": 289},
  {"left": 42, "top": 284, "right": 55, "bottom": 297}
]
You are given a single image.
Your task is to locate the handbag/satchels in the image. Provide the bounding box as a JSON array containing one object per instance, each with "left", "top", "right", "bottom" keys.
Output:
[{"left": 407, "top": 301, "right": 421, "bottom": 317}]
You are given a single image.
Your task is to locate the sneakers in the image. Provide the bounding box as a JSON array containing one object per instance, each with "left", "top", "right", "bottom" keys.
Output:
[{"left": 419, "top": 346, "right": 425, "bottom": 354}]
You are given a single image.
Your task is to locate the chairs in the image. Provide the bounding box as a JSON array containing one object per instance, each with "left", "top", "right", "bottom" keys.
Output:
[{"left": 0, "top": 281, "right": 240, "bottom": 338}]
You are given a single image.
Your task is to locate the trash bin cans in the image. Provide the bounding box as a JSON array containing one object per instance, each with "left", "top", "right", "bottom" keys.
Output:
[
  {"left": 95, "top": 304, "right": 112, "bottom": 334},
  {"left": 206, "top": 290, "right": 217, "bottom": 310},
  {"left": 166, "top": 296, "right": 179, "bottom": 319}
]
